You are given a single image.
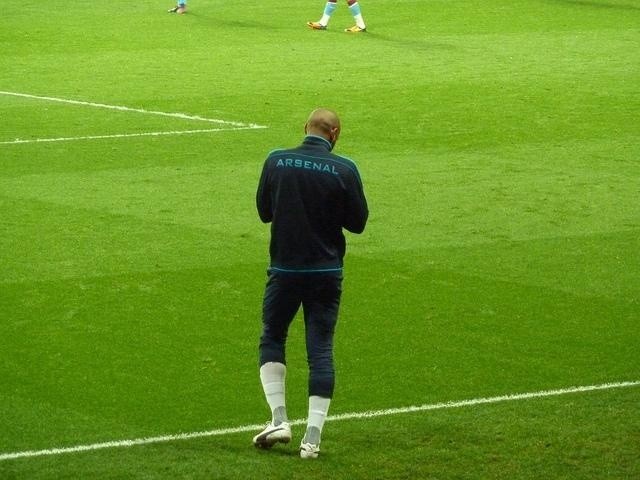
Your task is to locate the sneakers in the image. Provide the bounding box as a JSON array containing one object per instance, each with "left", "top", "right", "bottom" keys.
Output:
[
  {"left": 252, "top": 421, "right": 290, "bottom": 449},
  {"left": 301, "top": 438, "right": 320, "bottom": 459},
  {"left": 307, "top": 21, "right": 328, "bottom": 31},
  {"left": 168, "top": 6, "right": 185, "bottom": 13},
  {"left": 344, "top": 26, "right": 365, "bottom": 33}
]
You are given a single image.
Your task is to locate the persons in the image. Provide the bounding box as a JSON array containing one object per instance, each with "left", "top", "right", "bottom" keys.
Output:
[
  {"left": 307, "top": 0, "right": 367, "bottom": 32},
  {"left": 168, "top": 0, "right": 187, "bottom": 14},
  {"left": 253, "top": 108, "right": 369, "bottom": 459}
]
